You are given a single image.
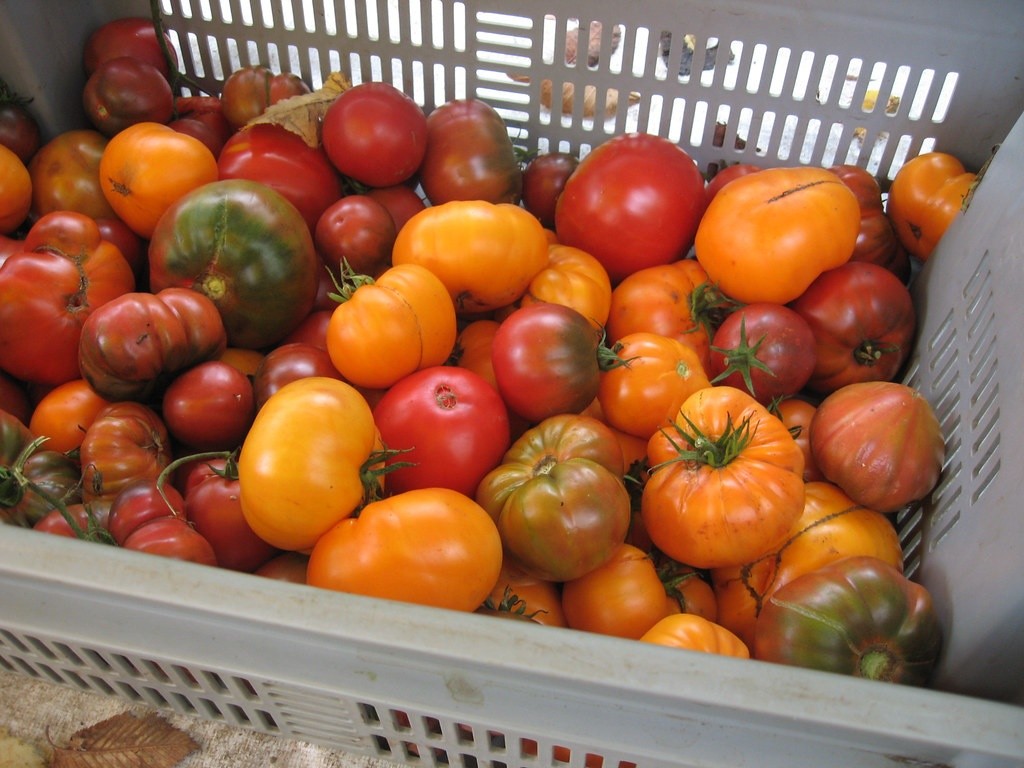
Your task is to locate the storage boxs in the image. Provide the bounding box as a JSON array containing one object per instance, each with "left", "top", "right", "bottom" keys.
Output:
[{"left": 0, "top": 0, "right": 1024, "bottom": 768}]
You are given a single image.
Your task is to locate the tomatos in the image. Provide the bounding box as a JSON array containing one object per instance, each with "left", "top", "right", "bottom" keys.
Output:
[{"left": 0, "top": 18, "right": 980, "bottom": 768}]
